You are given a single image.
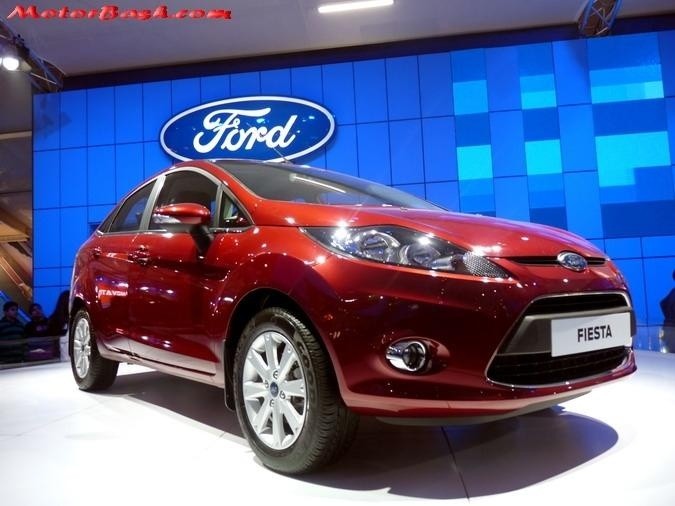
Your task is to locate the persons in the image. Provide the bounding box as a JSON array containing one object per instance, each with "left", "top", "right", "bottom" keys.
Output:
[
  {"left": 0, "top": 290, "right": 70, "bottom": 365},
  {"left": 659, "top": 269, "right": 675, "bottom": 354}
]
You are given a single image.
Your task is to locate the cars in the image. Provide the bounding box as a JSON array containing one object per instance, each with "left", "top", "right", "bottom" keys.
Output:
[{"left": 64, "top": 159, "right": 639, "bottom": 477}]
